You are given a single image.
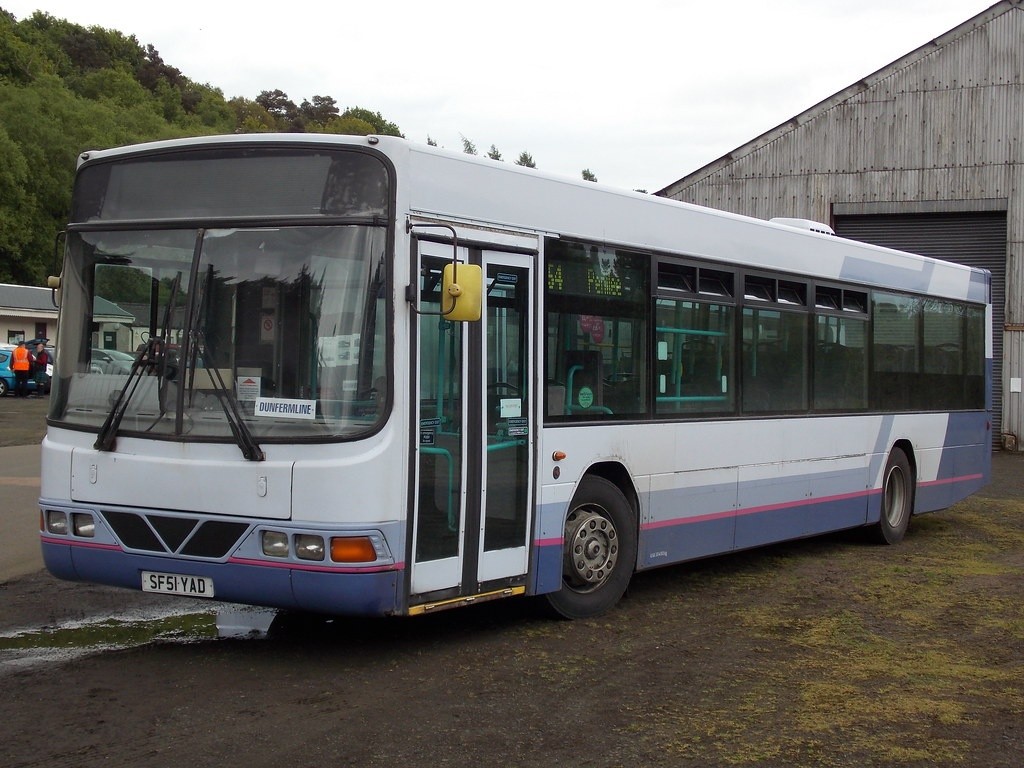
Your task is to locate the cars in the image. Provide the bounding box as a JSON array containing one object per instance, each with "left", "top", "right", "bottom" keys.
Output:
[{"left": 0, "top": 335, "right": 204, "bottom": 400}]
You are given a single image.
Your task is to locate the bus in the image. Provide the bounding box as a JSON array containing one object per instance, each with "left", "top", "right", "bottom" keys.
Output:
[{"left": 37, "top": 131, "right": 993, "bottom": 621}]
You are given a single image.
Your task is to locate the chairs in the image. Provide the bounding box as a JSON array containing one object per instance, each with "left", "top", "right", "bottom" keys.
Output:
[{"left": 615, "top": 339, "right": 980, "bottom": 396}]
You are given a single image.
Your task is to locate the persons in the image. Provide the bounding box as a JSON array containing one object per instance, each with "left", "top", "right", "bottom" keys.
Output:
[
  {"left": 9, "top": 341, "right": 34, "bottom": 399},
  {"left": 31, "top": 343, "right": 49, "bottom": 398}
]
